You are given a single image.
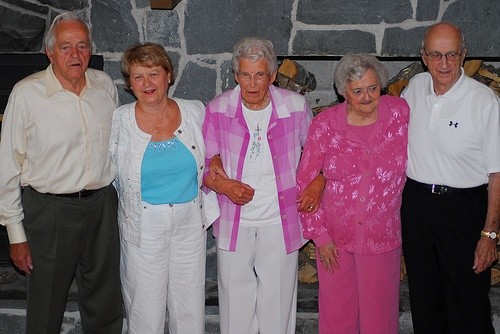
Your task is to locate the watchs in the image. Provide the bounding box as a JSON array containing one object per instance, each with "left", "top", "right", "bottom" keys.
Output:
[{"left": 481, "top": 231, "right": 500, "bottom": 241}]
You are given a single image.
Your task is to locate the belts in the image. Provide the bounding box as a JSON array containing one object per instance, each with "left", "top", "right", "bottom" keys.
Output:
[
  {"left": 53, "top": 189, "right": 97, "bottom": 200},
  {"left": 406, "top": 177, "right": 489, "bottom": 194}
]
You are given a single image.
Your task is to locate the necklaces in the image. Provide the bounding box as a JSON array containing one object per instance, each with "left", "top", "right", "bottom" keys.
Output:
[{"left": 143, "top": 109, "right": 160, "bottom": 135}]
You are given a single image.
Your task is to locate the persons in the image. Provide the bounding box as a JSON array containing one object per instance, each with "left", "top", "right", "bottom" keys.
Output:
[
  {"left": 400, "top": 21, "right": 500, "bottom": 334},
  {"left": 202, "top": 38, "right": 326, "bottom": 334},
  {"left": 296, "top": 50, "right": 411, "bottom": 334},
  {"left": 0, "top": 11, "right": 123, "bottom": 334},
  {"left": 108, "top": 43, "right": 229, "bottom": 334}
]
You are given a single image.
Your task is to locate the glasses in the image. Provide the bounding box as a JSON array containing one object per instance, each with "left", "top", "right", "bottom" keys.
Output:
[{"left": 424, "top": 48, "right": 463, "bottom": 61}]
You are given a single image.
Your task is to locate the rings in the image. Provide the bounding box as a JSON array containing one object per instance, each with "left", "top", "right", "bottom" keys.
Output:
[
  {"left": 321, "top": 258, "right": 323, "bottom": 262},
  {"left": 310, "top": 206, "right": 314, "bottom": 210}
]
